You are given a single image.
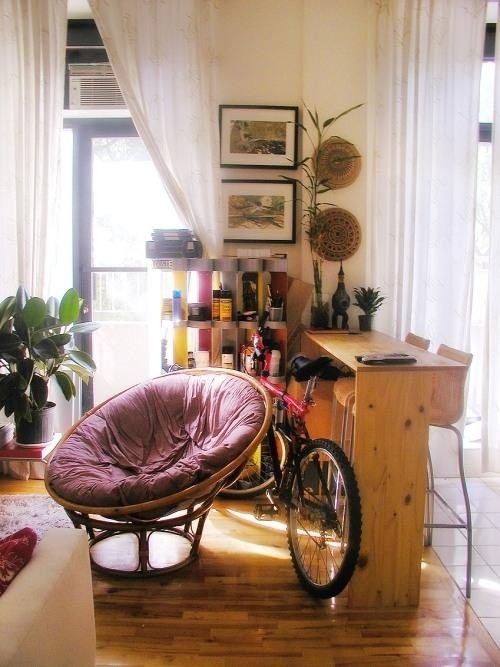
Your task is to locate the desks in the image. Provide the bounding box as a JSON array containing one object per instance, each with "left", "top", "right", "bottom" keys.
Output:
[{"left": 292, "top": 320, "right": 473, "bottom": 611}]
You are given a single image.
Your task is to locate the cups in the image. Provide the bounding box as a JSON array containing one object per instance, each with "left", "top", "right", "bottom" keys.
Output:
[
  {"left": 222, "top": 354, "right": 233, "bottom": 369},
  {"left": 196, "top": 350, "right": 210, "bottom": 367},
  {"left": 269, "top": 308, "right": 283, "bottom": 322}
]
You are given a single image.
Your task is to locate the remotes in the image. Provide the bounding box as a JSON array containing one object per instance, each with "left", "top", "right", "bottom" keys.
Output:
[{"left": 361, "top": 352, "right": 416, "bottom": 364}]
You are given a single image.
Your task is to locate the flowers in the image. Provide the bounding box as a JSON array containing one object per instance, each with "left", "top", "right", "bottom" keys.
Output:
[{"left": 278, "top": 100, "right": 379, "bottom": 310}]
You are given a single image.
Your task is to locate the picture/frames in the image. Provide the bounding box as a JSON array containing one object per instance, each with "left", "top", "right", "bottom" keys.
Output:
[
  {"left": 217, "top": 102, "right": 300, "bottom": 170},
  {"left": 219, "top": 177, "right": 298, "bottom": 245}
]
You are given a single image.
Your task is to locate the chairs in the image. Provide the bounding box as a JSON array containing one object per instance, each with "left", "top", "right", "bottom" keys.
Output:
[
  {"left": 321, "top": 332, "right": 431, "bottom": 555},
  {"left": 336, "top": 341, "right": 478, "bottom": 603},
  {"left": 0, "top": 525, "right": 98, "bottom": 666}
]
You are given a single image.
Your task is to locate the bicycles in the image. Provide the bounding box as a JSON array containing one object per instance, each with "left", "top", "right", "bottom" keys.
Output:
[{"left": 216, "top": 297, "right": 363, "bottom": 600}]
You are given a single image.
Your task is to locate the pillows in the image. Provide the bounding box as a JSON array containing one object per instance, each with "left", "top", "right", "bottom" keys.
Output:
[{"left": 0, "top": 523, "right": 40, "bottom": 600}]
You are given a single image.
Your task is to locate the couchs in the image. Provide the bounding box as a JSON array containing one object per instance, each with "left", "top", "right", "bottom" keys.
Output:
[{"left": 42, "top": 362, "right": 276, "bottom": 584}]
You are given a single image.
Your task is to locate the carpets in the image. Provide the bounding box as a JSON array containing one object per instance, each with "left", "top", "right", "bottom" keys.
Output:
[{"left": 0, "top": 493, "right": 93, "bottom": 541}]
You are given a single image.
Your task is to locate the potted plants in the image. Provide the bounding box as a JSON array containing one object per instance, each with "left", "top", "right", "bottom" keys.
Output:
[
  {"left": 0, "top": 285, "right": 103, "bottom": 448},
  {"left": 348, "top": 284, "right": 386, "bottom": 333}
]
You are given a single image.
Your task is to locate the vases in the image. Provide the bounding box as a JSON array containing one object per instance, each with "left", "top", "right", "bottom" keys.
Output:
[{"left": 308, "top": 289, "right": 334, "bottom": 333}]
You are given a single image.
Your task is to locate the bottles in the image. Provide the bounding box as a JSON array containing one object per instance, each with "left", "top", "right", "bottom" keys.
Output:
[
  {"left": 275, "top": 401, "right": 289, "bottom": 431},
  {"left": 269, "top": 343, "right": 281, "bottom": 377},
  {"left": 172, "top": 289, "right": 183, "bottom": 321},
  {"left": 219, "top": 290, "right": 232, "bottom": 321},
  {"left": 187, "top": 351, "right": 196, "bottom": 368},
  {"left": 212, "top": 290, "right": 221, "bottom": 320}
]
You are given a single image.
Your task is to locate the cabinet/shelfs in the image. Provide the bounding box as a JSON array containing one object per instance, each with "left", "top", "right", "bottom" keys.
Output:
[{"left": 153, "top": 249, "right": 296, "bottom": 505}]
